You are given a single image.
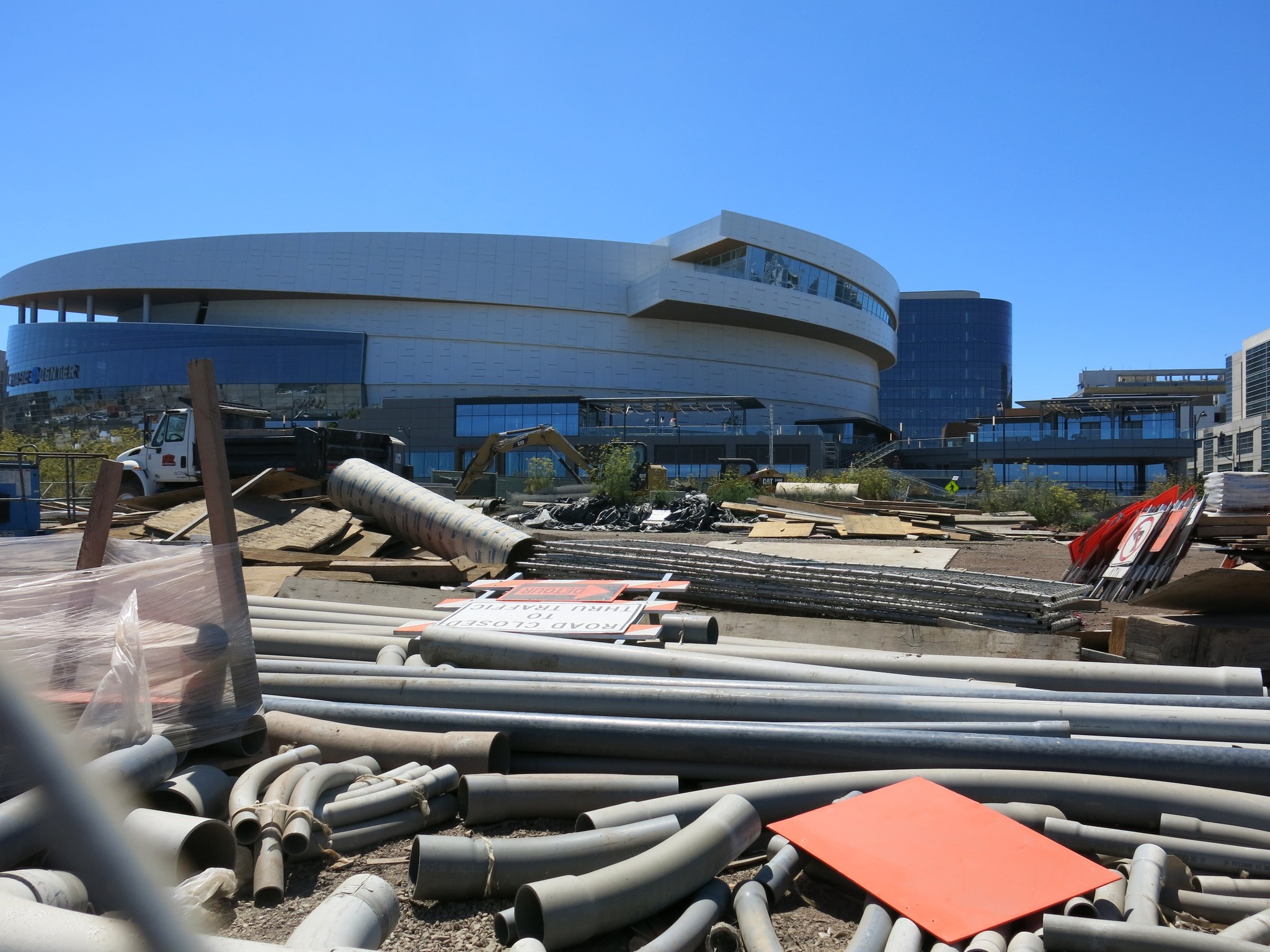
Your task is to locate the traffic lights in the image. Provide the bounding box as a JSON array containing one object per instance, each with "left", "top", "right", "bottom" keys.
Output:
[
  {"left": 1236, "top": 466, "right": 1242, "bottom": 472},
  {"left": 1219, "top": 432, "right": 1226, "bottom": 446}
]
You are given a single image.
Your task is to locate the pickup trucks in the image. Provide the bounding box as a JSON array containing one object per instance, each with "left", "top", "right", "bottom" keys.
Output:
[{"left": 115, "top": 407, "right": 393, "bottom": 514}]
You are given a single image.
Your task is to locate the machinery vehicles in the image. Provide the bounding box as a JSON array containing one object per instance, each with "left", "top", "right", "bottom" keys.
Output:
[
  {"left": 455, "top": 424, "right": 670, "bottom": 507},
  {"left": 706, "top": 457, "right": 787, "bottom": 503}
]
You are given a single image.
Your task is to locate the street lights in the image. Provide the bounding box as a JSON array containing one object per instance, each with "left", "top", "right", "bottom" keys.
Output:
[
  {"left": 73, "top": 411, "right": 99, "bottom": 453},
  {"left": 1238, "top": 424, "right": 1270, "bottom": 472},
  {"left": 996, "top": 401, "right": 1007, "bottom": 499},
  {"left": 1214, "top": 451, "right": 1234, "bottom": 472},
  {"left": 1193, "top": 411, "right": 1208, "bottom": 492},
  {"left": 975, "top": 421, "right": 983, "bottom": 498},
  {"left": 398, "top": 425, "right": 411, "bottom": 466}
]
